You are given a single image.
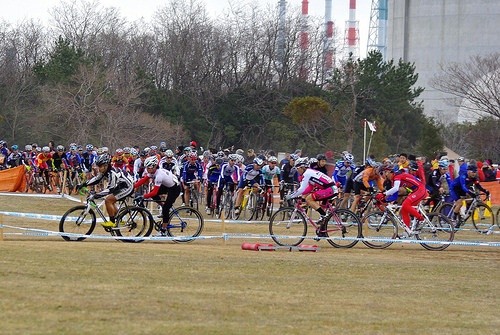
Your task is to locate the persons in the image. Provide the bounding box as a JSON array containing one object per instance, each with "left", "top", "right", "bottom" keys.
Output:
[
  {"left": 133, "top": 156, "right": 181, "bottom": 236},
  {"left": 74, "top": 153, "right": 133, "bottom": 227},
  {"left": 0, "top": 140, "right": 497, "bottom": 225},
  {"left": 284, "top": 157, "right": 338, "bottom": 239},
  {"left": 450, "top": 165, "right": 489, "bottom": 222},
  {"left": 374, "top": 163, "right": 426, "bottom": 238}
]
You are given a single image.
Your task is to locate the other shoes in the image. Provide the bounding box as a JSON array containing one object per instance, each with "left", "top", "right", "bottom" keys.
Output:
[
  {"left": 157, "top": 212, "right": 164, "bottom": 218},
  {"left": 101, "top": 221, "right": 117, "bottom": 228},
  {"left": 181, "top": 202, "right": 185, "bottom": 206},
  {"left": 453, "top": 213, "right": 458, "bottom": 219},
  {"left": 317, "top": 214, "right": 329, "bottom": 224},
  {"left": 48, "top": 185, "right": 52, "bottom": 190},
  {"left": 416, "top": 220, "right": 426, "bottom": 230},
  {"left": 161, "top": 227, "right": 168, "bottom": 236},
  {"left": 115, "top": 221, "right": 125, "bottom": 226},
  {"left": 57, "top": 187, "right": 60, "bottom": 193},
  {"left": 234, "top": 206, "right": 241, "bottom": 214},
  {"left": 207, "top": 208, "right": 211, "bottom": 215}
]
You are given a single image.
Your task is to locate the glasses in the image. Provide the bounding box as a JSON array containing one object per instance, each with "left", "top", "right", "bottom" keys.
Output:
[{"left": 380, "top": 171, "right": 383, "bottom": 175}]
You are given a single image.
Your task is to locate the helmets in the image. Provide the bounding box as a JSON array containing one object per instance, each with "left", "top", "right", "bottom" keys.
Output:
[
  {"left": 387, "top": 164, "right": 399, "bottom": 171},
  {"left": 439, "top": 161, "right": 448, "bottom": 169},
  {"left": 0, "top": 141, "right": 354, "bottom": 168},
  {"left": 467, "top": 165, "right": 477, "bottom": 171},
  {"left": 457, "top": 157, "right": 464, "bottom": 162}
]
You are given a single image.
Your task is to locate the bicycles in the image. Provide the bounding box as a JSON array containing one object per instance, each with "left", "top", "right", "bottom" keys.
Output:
[
  {"left": 438, "top": 190, "right": 494, "bottom": 234},
  {"left": 336, "top": 189, "right": 389, "bottom": 227},
  {"left": 115, "top": 192, "right": 204, "bottom": 243},
  {"left": 269, "top": 192, "right": 362, "bottom": 248},
  {"left": 58, "top": 186, "right": 154, "bottom": 243},
  {"left": 185, "top": 180, "right": 299, "bottom": 221},
  {"left": 358, "top": 190, "right": 454, "bottom": 251},
  {"left": 26, "top": 168, "right": 83, "bottom": 195},
  {"left": 496, "top": 208, "right": 500, "bottom": 229}
]
